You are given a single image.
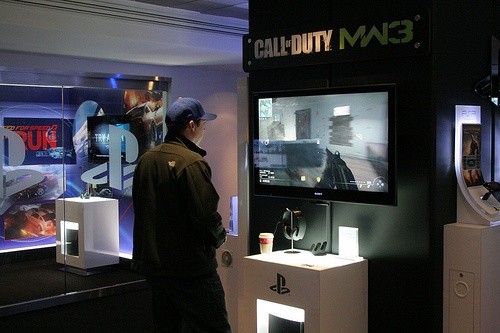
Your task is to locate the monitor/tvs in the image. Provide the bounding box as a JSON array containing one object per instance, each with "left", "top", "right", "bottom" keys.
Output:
[
  {"left": 247, "top": 83, "right": 398, "bottom": 207},
  {"left": 87, "top": 115, "right": 145, "bottom": 163}
]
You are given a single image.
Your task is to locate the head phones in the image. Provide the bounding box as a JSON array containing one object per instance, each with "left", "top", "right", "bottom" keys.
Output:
[
  {"left": 81, "top": 189, "right": 90, "bottom": 199},
  {"left": 281, "top": 210, "right": 306, "bottom": 241}
]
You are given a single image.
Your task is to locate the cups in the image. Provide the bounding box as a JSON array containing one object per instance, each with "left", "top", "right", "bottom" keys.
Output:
[{"left": 259, "top": 232, "right": 274, "bottom": 254}]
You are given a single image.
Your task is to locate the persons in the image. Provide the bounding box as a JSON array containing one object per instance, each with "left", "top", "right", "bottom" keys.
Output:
[{"left": 130, "top": 97, "right": 233, "bottom": 333}]
